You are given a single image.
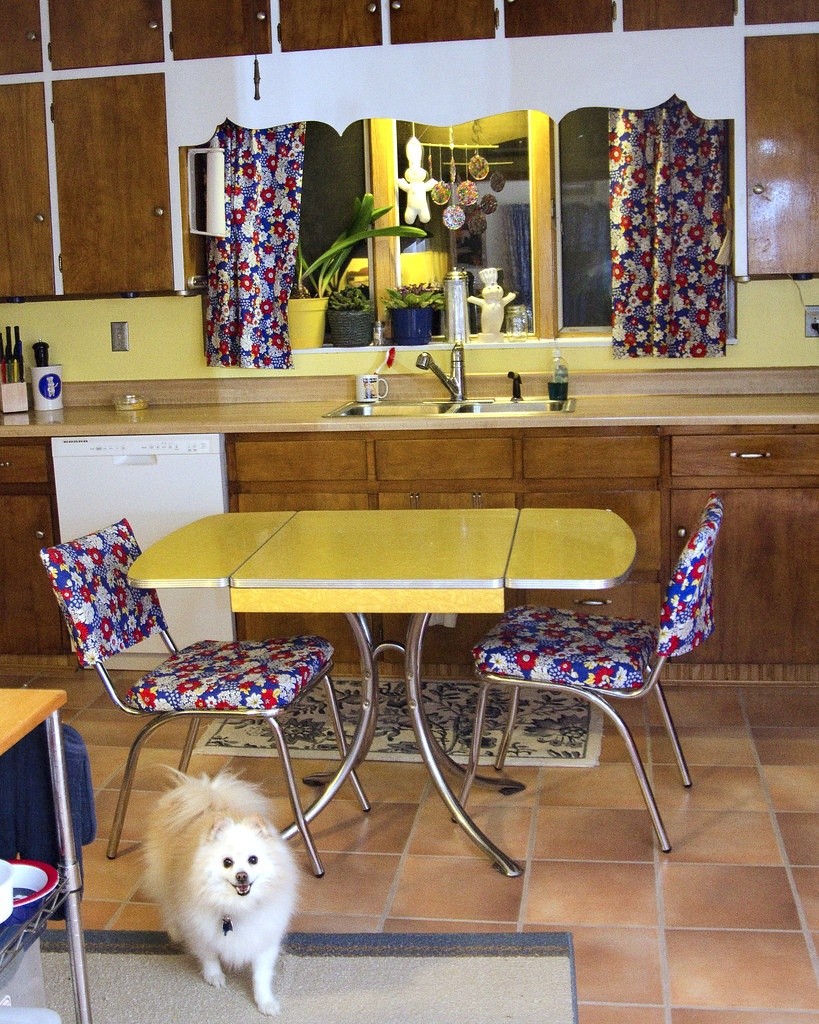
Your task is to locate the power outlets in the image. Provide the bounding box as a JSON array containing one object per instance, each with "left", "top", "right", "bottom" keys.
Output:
[
  {"left": 804, "top": 305, "right": 819, "bottom": 338},
  {"left": 109, "top": 320, "right": 134, "bottom": 353}
]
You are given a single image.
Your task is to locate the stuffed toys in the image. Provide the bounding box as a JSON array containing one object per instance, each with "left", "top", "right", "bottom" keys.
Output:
[{"left": 398, "top": 137, "right": 437, "bottom": 224}]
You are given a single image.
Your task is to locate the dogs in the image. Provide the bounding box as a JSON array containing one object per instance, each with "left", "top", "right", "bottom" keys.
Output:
[{"left": 129, "top": 762, "right": 304, "bottom": 1018}]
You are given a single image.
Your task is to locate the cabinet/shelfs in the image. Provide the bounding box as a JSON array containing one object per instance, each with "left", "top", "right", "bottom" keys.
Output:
[
  {"left": 1, "top": 0, "right": 819, "bottom": 303},
  {"left": 0, "top": 421, "right": 818, "bottom": 699}
]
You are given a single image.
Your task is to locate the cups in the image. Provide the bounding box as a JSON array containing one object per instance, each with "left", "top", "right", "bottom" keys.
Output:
[
  {"left": 30, "top": 366, "right": 63, "bottom": 410},
  {"left": 0, "top": 860, "right": 13, "bottom": 924},
  {"left": 356, "top": 375, "right": 388, "bottom": 402}
]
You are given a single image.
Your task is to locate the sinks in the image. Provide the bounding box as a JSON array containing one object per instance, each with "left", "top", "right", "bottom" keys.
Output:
[
  {"left": 455, "top": 400, "right": 564, "bottom": 414},
  {"left": 341, "top": 403, "right": 455, "bottom": 416}
]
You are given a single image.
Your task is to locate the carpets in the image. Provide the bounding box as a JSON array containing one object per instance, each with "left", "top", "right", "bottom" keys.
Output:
[
  {"left": 194, "top": 669, "right": 605, "bottom": 768},
  {"left": 36, "top": 927, "right": 580, "bottom": 1024}
]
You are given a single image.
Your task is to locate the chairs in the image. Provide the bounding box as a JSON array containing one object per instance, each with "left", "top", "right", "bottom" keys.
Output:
[
  {"left": 36, "top": 517, "right": 372, "bottom": 879},
  {"left": 451, "top": 494, "right": 724, "bottom": 856}
]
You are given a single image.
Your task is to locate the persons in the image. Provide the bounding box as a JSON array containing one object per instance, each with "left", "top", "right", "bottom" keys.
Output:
[{"left": 467, "top": 268, "right": 516, "bottom": 332}]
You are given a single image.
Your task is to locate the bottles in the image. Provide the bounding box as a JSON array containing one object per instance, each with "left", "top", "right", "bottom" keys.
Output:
[
  {"left": 507, "top": 306, "right": 528, "bottom": 340},
  {"left": 443, "top": 267, "right": 471, "bottom": 344}
]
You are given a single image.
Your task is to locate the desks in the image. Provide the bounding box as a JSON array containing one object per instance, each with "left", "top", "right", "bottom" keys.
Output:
[
  {"left": 126, "top": 510, "right": 639, "bottom": 881},
  {"left": 0, "top": 686, "right": 100, "bottom": 1024}
]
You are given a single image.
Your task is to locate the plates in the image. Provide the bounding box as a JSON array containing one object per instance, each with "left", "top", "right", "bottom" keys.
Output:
[{"left": 8, "top": 861, "right": 58, "bottom": 907}]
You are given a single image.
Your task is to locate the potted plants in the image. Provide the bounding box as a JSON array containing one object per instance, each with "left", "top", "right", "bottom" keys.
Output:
[
  {"left": 287, "top": 193, "right": 429, "bottom": 347},
  {"left": 326, "top": 287, "right": 375, "bottom": 347},
  {"left": 381, "top": 284, "right": 445, "bottom": 345}
]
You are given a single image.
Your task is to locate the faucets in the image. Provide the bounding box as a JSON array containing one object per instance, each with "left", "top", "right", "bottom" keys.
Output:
[{"left": 415, "top": 340, "right": 466, "bottom": 401}]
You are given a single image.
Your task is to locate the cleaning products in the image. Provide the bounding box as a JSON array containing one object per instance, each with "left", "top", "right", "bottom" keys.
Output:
[{"left": 546, "top": 345, "right": 570, "bottom": 401}]
